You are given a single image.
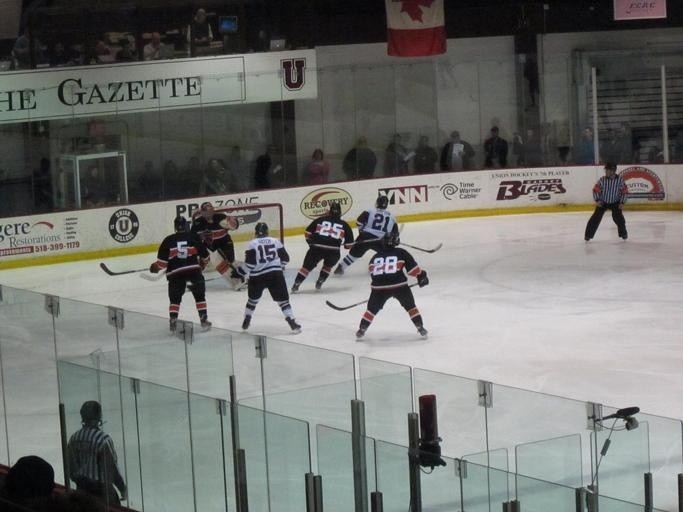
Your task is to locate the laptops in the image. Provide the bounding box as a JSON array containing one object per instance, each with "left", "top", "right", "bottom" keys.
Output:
[{"left": 268, "top": 38, "right": 286, "bottom": 50}]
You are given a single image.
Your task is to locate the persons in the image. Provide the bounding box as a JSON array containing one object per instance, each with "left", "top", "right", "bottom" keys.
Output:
[
  {"left": 191, "top": 202, "right": 248, "bottom": 291},
  {"left": 352, "top": 233, "right": 429, "bottom": 338},
  {"left": 584, "top": 163, "right": 628, "bottom": 241},
  {"left": 1, "top": 456, "right": 62, "bottom": 511},
  {"left": 2, "top": 9, "right": 321, "bottom": 70},
  {"left": 231, "top": 222, "right": 301, "bottom": 330},
  {"left": 33, "top": 122, "right": 683, "bottom": 204},
  {"left": 293, "top": 203, "right": 354, "bottom": 290},
  {"left": 67, "top": 400, "right": 127, "bottom": 508},
  {"left": 334, "top": 195, "right": 400, "bottom": 273},
  {"left": 150, "top": 215, "right": 212, "bottom": 329}
]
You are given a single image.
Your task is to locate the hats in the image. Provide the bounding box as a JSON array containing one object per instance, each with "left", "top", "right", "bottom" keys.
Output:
[{"left": 9, "top": 456, "right": 54, "bottom": 499}]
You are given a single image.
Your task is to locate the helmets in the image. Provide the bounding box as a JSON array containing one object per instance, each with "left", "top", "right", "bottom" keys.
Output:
[
  {"left": 379, "top": 232, "right": 397, "bottom": 250},
  {"left": 200, "top": 202, "right": 213, "bottom": 219},
  {"left": 254, "top": 222, "right": 268, "bottom": 236},
  {"left": 329, "top": 203, "right": 341, "bottom": 217},
  {"left": 173, "top": 216, "right": 186, "bottom": 232},
  {"left": 604, "top": 161, "right": 616, "bottom": 170},
  {"left": 375, "top": 196, "right": 388, "bottom": 208}
]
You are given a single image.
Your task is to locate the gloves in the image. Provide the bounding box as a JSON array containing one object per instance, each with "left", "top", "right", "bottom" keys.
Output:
[
  {"left": 343, "top": 241, "right": 353, "bottom": 248},
  {"left": 392, "top": 235, "right": 399, "bottom": 246},
  {"left": 199, "top": 257, "right": 209, "bottom": 270},
  {"left": 416, "top": 269, "right": 429, "bottom": 287},
  {"left": 149, "top": 262, "right": 161, "bottom": 274},
  {"left": 229, "top": 270, "right": 242, "bottom": 279}
]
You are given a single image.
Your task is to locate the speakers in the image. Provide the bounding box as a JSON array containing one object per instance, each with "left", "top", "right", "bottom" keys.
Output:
[{"left": 418, "top": 395, "right": 440, "bottom": 466}]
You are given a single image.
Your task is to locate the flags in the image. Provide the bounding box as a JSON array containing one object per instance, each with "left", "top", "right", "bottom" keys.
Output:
[{"left": 382, "top": 0, "right": 447, "bottom": 57}]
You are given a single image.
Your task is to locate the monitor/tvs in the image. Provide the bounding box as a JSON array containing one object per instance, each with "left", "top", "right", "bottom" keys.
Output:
[{"left": 217, "top": 14, "right": 238, "bottom": 34}]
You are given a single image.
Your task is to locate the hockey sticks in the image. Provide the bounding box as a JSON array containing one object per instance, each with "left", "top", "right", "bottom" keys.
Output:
[
  {"left": 98, "top": 262, "right": 151, "bottom": 277},
  {"left": 139, "top": 267, "right": 168, "bottom": 282},
  {"left": 326, "top": 282, "right": 421, "bottom": 311},
  {"left": 398, "top": 239, "right": 444, "bottom": 254}
]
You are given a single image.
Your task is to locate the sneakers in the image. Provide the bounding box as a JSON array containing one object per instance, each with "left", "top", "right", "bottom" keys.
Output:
[
  {"left": 241, "top": 318, "right": 249, "bottom": 331},
  {"left": 334, "top": 270, "right": 345, "bottom": 277},
  {"left": 619, "top": 232, "right": 628, "bottom": 241},
  {"left": 168, "top": 318, "right": 177, "bottom": 335},
  {"left": 287, "top": 319, "right": 301, "bottom": 334},
  {"left": 355, "top": 327, "right": 367, "bottom": 341},
  {"left": 416, "top": 327, "right": 429, "bottom": 340},
  {"left": 314, "top": 279, "right": 323, "bottom": 289},
  {"left": 290, "top": 284, "right": 300, "bottom": 294},
  {"left": 584, "top": 235, "right": 591, "bottom": 244},
  {"left": 200, "top": 319, "right": 211, "bottom": 332}
]
